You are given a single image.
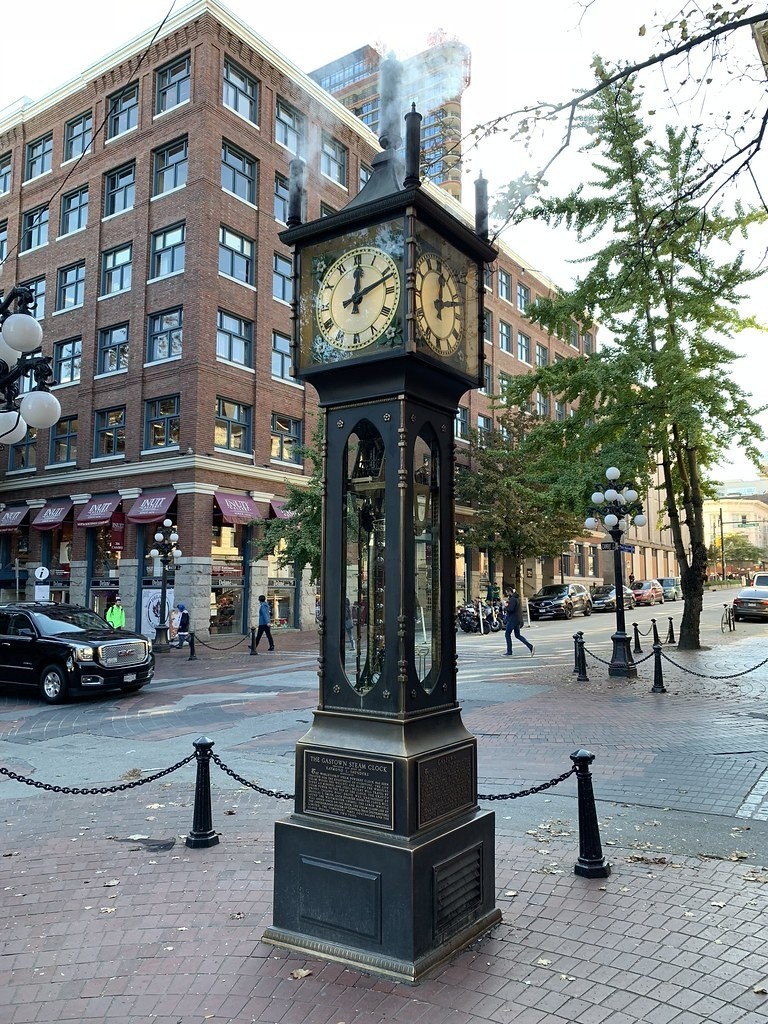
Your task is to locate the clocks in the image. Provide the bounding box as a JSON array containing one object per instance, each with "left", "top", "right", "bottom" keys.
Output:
[
  {"left": 312, "top": 229, "right": 403, "bottom": 367},
  {"left": 414, "top": 234, "right": 467, "bottom": 374}
]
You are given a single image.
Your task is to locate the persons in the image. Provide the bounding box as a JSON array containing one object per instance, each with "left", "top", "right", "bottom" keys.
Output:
[
  {"left": 344, "top": 598, "right": 355, "bottom": 650},
  {"left": 176, "top": 604, "right": 190, "bottom": 649},
  {"left": 629, "top": 573, "right": 635, "bottom": 585},
  {"left": 502, "top": 585, "right": 535, "bottom": 657},
  {"left": 726, "top": 572, "right": 733, "bottom": 580},
  {"left": 106, "top": 599, "right": 125, "bottom": 631},
  {"left": 248, "top": 595, "right": 274, "bottom": 651},
  {"left": 486, "top": 581, "right": 500, "bottom": 602},
  {"left": 704, "top": 572, "right": 719, "bottom": 582}
]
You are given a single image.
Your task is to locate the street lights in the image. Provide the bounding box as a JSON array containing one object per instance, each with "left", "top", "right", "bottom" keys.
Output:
[
  {"left": 150, "top": 518, "right": 181, "bottom": 650},
  {"left": 688, "top": 543, "right": 694, "bottom": 567},
  {"left": 583, "top": 465, "right": 646, "bottom": 678}
]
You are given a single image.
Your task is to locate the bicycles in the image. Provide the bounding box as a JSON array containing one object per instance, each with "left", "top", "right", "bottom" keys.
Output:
[{"left": 721, "top": 603, "right": 738, "bottom": 633}]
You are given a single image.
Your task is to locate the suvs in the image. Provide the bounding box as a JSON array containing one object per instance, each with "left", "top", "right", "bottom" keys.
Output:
[{"left": 0, "top": 601, "right": 156, "bottom": 703}]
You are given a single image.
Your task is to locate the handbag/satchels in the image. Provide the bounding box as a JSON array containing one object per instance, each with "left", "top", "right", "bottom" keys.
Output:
[{"left": 345, "top": 618, "right": 353, "bottom": 630}]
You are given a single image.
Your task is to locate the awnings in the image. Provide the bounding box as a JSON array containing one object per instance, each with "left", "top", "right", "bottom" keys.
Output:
[
  {"left": 0, "top": 506, "right": 30, "bottom": 532},
  {"left": 271, "top": 500, "right": 302, "bottom": 520},
  {"left": 31, "top": 499, "right": 73, "bottom": 531},
  {"left": 215, "top": 493, "right": 261, "bottom": 525},
  {"left": 75, "top": 493, "right": 121, "bottom": 527},
  {"left": 127, "top": 491, "right": 176, "bottom": 525}
]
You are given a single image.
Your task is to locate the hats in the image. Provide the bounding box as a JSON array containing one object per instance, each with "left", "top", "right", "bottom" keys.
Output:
[{"left": 177, "top": 604, "right": 185, "bottom": 612}]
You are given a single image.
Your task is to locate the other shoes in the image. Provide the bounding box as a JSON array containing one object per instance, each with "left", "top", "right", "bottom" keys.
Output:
[
  {"left": 248, "top": 646, "right": 252, "bottom": 649},
  {"left": 531, "top": 646, "right": 535, "bottom": 657},
  {"left": 175, "top": 645, "right": 182, "bottom": 649},
  {"left": 268, "top": 645, "right": 274, "bottom": 651},
  {"left": 502, "top": 652, "right": 512, "bottom": 657}
]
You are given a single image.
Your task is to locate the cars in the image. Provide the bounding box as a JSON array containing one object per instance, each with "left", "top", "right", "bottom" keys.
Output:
[
  {"left": 590, "top": 585, "right": 637, "bottom": 613},
  {"left": 627, "top": 579, "right": 665, "bottom": 606},
  {"left": 456, "top": 596, "right": 509, "bottom": 634},
  {"left": 657, "top": 577, "right": 686, "bottom": 600},
  {"left": 732, "top": 587, "right": 768, "bottom": 621},
  {"left": 528, "top": 583, "right": 593, "bottom": 621}
]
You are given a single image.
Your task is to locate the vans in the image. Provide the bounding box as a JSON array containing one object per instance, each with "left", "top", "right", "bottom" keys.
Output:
[{"left": 750, "top": 572, "right": 768, "bottom": 587}]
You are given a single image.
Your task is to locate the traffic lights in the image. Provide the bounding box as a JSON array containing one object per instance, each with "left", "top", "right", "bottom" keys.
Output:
[{"left": 742, "top": 514, "right": 747, "bottom": 525}]
